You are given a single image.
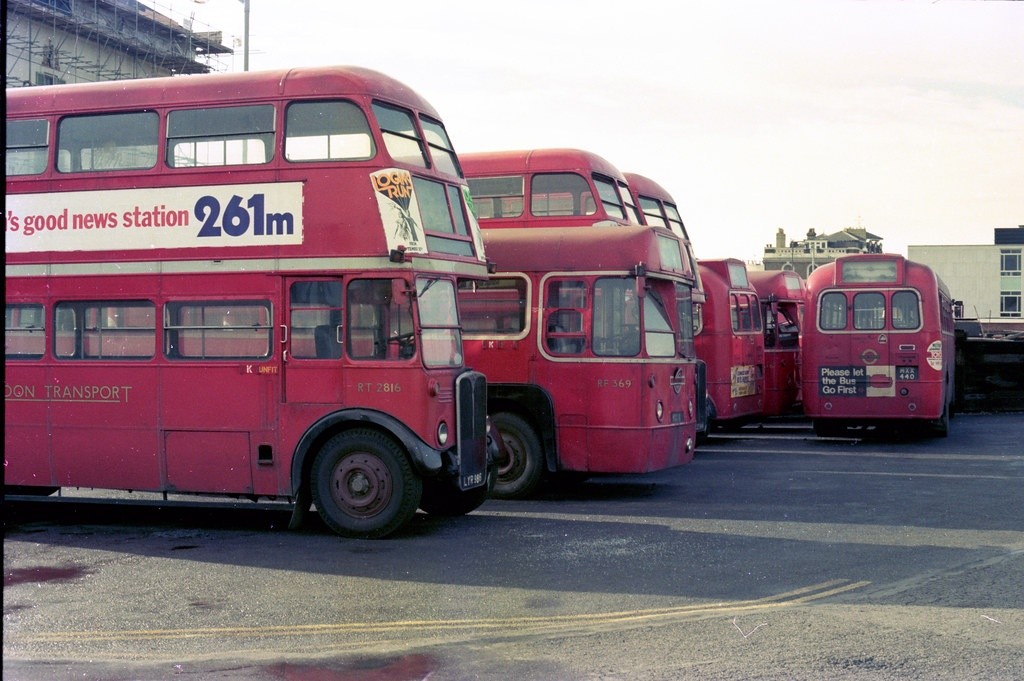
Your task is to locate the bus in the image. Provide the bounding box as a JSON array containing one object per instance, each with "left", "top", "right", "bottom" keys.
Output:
[
  {"left": 745, "top": 267, "right": 808, "bottom": 418},
  {"left": 13, "top": 147, "right": 719, "bottom": 499},
  {"left": 459, "top": 257, "right": 768, "bottom": 444},
  {"left": 6, "top": 62, "right": 498, "bottom": 541},
  {"left": 800, "top": 251, "right": 964, "bottom": 439}
]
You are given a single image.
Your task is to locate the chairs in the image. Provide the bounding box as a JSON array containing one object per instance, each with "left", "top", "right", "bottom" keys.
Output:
[{"left": 314, "top": 324, "right": 341, "bottom": 360}]
